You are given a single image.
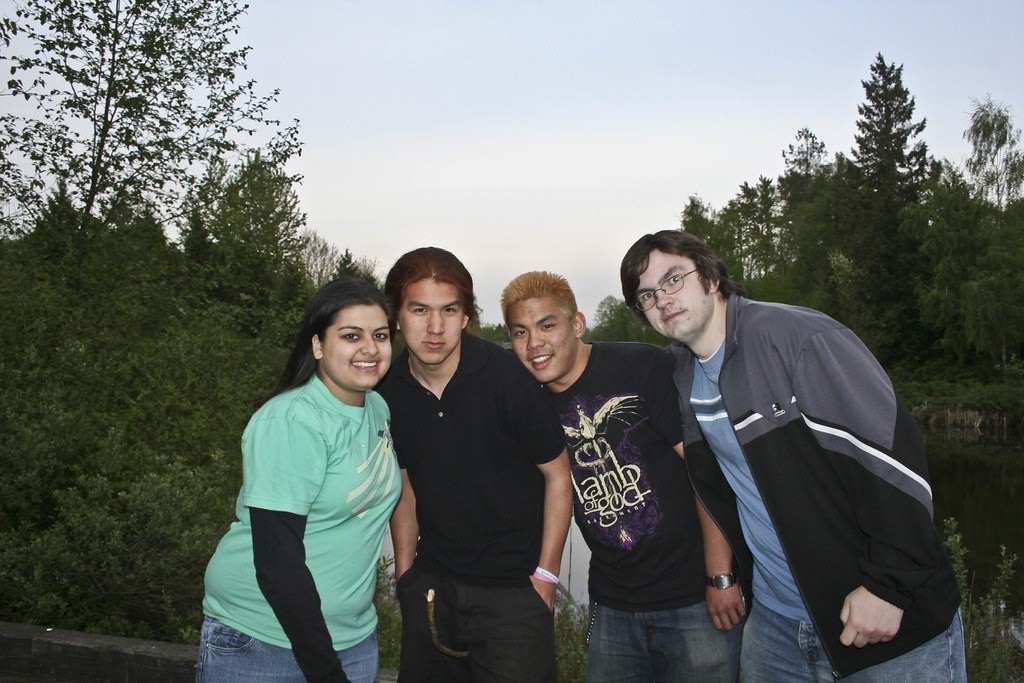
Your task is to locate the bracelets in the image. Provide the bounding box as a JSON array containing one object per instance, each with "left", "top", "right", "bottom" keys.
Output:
[
  {"left": 704, "top": 572, "right": 736, "bottom": 590},
  {"left": 534, "top": 567, "right": 559, "bottom": 586}
]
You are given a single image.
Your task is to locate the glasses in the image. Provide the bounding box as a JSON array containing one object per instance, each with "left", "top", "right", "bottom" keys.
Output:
[{"left": 635, "top": 268, "right": 697, "bottom": 311}]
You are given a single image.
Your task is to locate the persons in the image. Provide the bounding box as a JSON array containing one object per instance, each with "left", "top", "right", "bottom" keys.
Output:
[
  {"left": 500, "top": 269, "right": 749, "bottom": 682},
  {"left": 197, "top": 275, "right": 404, "bottom": 683},
  {"left": 620, "top": 229, "right": 970, "bottom": 683},
  {"left": 371, "top": 246, "right": 574, "bottom": 683}
]
